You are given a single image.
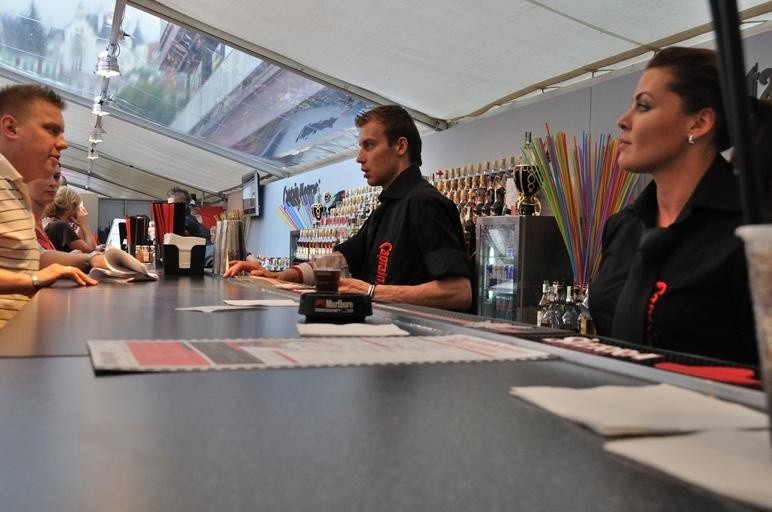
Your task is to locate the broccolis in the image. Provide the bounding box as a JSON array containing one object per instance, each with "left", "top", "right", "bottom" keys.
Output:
[{"left": 474, "top": 215, "right": 574, "bottom": 324}]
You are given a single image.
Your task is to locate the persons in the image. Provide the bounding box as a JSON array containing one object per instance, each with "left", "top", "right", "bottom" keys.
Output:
[
  {"left": 41, "top": 182, "right": 97, "bottom": 253},
  {"left": 152, "top": 186, "right": 200, "bottom": 245},
  {"left": 582, "top": 44, "right": 766, "bottom": 375},
  {"left": 28, "top": 154, "right": 60, "bottom": 251},
  {"left": 1, "top": 83, "right": 99, "bottom": 332},
  {"left": 219, "top": 102, "right": 479, "bottom": 315}
]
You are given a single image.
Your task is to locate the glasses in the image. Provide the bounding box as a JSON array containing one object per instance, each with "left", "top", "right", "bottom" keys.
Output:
[{"left": 85, "top": 43, "right": 121, "bottom": 161}]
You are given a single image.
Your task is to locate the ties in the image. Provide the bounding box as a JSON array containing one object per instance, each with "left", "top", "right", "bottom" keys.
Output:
[{"left": 242, "top": 170, "right": 260, "bottom": 216}]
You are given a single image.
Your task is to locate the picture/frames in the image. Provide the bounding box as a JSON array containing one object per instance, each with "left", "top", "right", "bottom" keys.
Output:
[{"left": 0, "top": 268, "right": 770, "bottom": 512}]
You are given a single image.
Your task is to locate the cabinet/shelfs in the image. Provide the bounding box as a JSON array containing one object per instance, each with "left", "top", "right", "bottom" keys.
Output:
[
  {"left": 135, "top": 245, "right": 156, "bottom": 263},
  {"left": 296, "top": 131, "right": 534, "bottom": 263},
  {"left": 536, "top": 279, "right": 595, "bottom": 334}
]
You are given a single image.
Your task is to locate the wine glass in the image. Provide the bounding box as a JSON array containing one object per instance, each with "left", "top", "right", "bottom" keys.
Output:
[{"left": 68, "top": 265, "right": 72, "bottom": 271}]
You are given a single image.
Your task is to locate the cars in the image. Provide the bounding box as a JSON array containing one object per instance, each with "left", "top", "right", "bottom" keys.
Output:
[{"left": 311, "top": 253, "right": 345, "bottom": 294}]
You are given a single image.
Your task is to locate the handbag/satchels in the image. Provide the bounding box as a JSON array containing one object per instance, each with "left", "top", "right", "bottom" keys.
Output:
[
  {"left": 25, "top": 270, "right": 39, "bottom": 297},
  {"left": 367, "top": 284, "right": 375, "bottom": 298}
]
